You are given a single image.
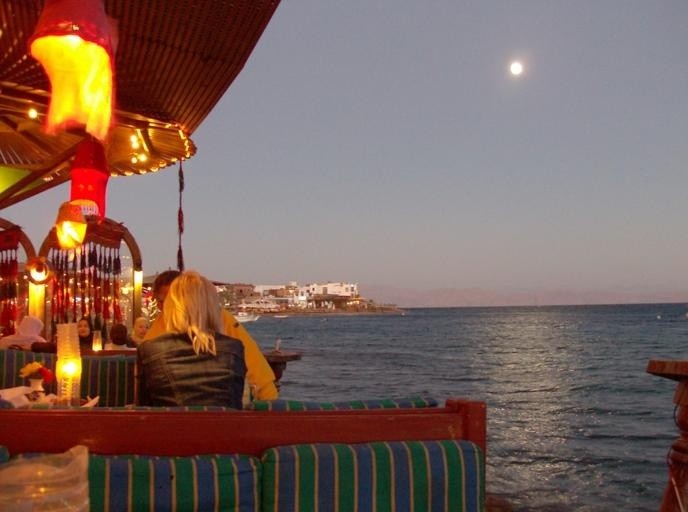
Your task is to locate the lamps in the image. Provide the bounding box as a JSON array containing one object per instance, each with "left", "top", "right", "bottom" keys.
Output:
[{"left": 53, "top": 319, "right": 84, "bottom": 410}]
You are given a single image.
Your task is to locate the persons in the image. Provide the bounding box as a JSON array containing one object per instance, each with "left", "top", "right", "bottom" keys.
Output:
[
  {"left": 137, "top": 269, "right": 248, "bottom": 410},
  {"left": 144, "top": 271, "right": 279, "bottom": 401},
  {"left": 0, "top": 315, "right": 149, "bottom": 353}
]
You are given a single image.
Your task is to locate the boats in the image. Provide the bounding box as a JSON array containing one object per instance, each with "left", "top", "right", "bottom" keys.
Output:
[
  {"left": 233, "top": 310, "right": 260, "bottom": 323},
  {"left": 273, "top": 314, "right": 288, "bottom": 319}
]
